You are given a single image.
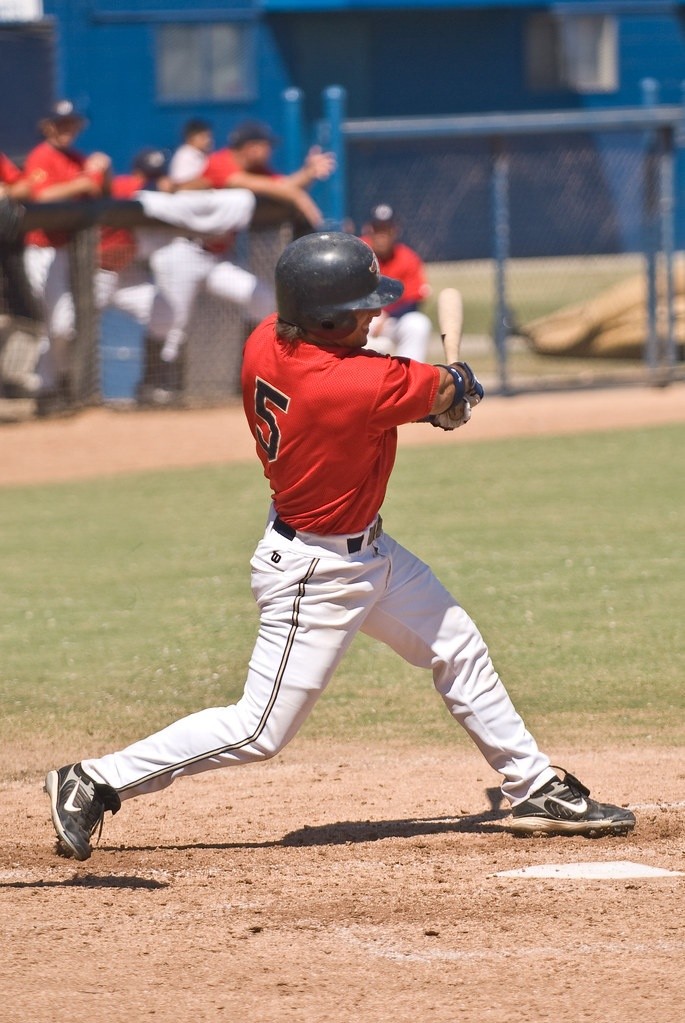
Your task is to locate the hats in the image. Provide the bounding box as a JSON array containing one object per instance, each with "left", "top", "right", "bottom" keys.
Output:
[
  {"left": 47, "top": 98, "right": 89, "bottom": 129},
  {"left": 138, "top": 149, "right": 171, "bottom": 177},
  {"left": 232, "top": 122, "right": 276, "bottom": 142},
  {"left": 370, "top": 205, "right": 396, "bottom": 228}
]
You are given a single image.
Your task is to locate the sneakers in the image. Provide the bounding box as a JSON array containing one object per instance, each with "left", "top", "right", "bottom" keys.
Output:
[
  {"left": 512, "top": 765, "right": 636, "bottom": 832},
  {"left": 43, "top": 763, "right": 121, "bottom": 862}
]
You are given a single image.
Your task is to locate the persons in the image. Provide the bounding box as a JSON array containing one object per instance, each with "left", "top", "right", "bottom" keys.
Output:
[
  {"left": 46, "top": 232, "right": 636, "bottom": 862},
  {"left": 357, "top": 203, "right": 432, "bottom": 362},
  {"left": 0, "top": 98, "right": 335, "bottom": 395}
]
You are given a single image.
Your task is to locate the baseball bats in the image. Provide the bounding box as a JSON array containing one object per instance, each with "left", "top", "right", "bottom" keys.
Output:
[{"left": 437, "top": 286, "right": 467, "bottom": 422}]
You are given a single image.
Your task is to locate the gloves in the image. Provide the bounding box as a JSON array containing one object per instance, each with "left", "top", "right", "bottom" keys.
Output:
[{"left": 412, "top": 362, "right": 484, "bottom": 432}]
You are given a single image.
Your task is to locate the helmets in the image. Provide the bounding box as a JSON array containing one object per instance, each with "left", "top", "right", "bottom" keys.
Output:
[{"left": 276, "top": 232, "right": 404, "bottom": 340}]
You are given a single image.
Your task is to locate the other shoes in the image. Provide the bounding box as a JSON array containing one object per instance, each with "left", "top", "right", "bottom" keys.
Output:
[{"left": 37, "top": 391, "right": 78, "bottom": 419}]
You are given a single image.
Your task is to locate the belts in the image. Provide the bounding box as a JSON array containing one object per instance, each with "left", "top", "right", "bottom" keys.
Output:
[{"left": 272, "top": 512, "right": 383, "bottom": 554}]
